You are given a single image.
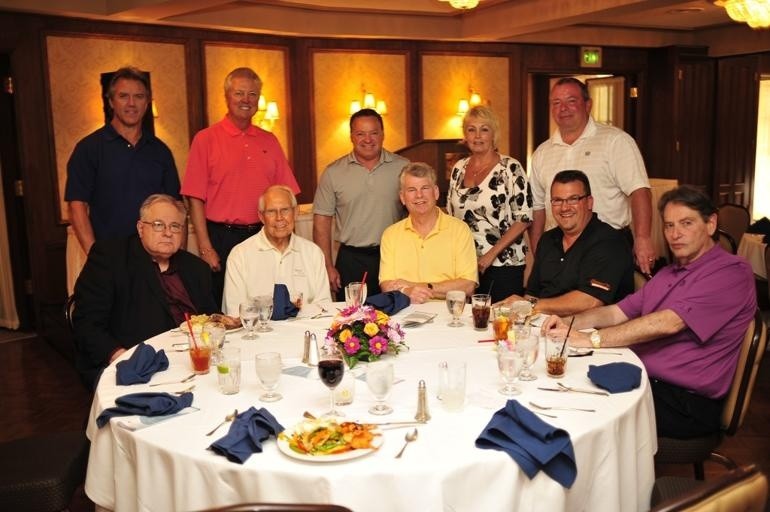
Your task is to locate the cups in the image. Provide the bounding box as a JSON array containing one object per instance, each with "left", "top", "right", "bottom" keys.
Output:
[
  {"left": 335, "top": 370, "right": 356, "bottom": 406},
  {"left": 349, "top": 282, "right": 367, "bottom": 305},
  {"left": 494, "top": 305, "right": 514, "bottom": 345},
  {"left": 216, "top": 347, "right": 241, "bottom": 394},
  {"left": 471, "top": 294, "right": 491, "bottom": 330},
  {"left": 513, "top": 301, "right": 532, "bottom": 330},
  {"left": 545, "top": 332, "right": 571, "bottom": 377},
  {"left": 188, "top": 330, "right": 212, "bottom": 374},
  {"left": 437, "top": 360, "right": 468, "bottom": 412},
  {"left": 344, "top": 286, "right": 361, "bottom": 307}
]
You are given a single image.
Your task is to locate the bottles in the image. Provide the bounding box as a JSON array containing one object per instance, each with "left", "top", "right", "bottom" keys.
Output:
[
  {"left": 309, "top": 333, "right": 320, "bottom": 367},
  {"left": 415, "top": 380, "right": 431, "bottom": 422},
  {"left": 302, "top": 331, "right": 312, "bottom": 363}
]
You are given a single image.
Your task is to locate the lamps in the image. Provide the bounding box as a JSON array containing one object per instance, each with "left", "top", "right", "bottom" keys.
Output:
[
  {"left": 455, "top": 86, "right": 483, "bottom": 115},
  {"left": 348, "top": 94, "right": 387, "bottom": 117},
  {"left": 257, "top": 96, "right": 280, "bottom": 132},
  {"left": 709, "top": 0, "right": 770, "bottom": 31},
  {"left": 443, "top": 0, "right": 481, "bottom": 9}
]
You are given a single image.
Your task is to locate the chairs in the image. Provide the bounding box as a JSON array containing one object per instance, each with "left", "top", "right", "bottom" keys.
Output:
[
  {"left": 717, "top": 203, "right": 749, "bottom": 244},
  {"left": 648, "top": 460, "right": 770, "bottom": 512},
  {"left": 197, "top": 503, "right": 353, "bottom": 512},
  {"left": 63, "top": 292, "right": 126, "bottom": 391},
  {"left": 652, "top": 304, "right": 768, "bottom": 478},
  {"left": 715, "top": 227, "right": 736, "bottom": 256}
]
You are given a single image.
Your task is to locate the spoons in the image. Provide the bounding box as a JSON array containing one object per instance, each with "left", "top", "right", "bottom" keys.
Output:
[
  {"left": 205, "top": 408, "right": 238, "bottom": 436},
  {"left": 393, "top": 427, "right": 418, "bottom": 459}
]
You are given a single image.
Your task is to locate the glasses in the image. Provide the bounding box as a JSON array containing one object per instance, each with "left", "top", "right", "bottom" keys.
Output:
[
  {"left": 550, "top": 194, "right": 587, "bottom": 204},
  {"left": 141, "top": 221, "right": 185, "bottom": 231},
  {"left": 230, "top": 90, "right": 260, "bottom": 98}
]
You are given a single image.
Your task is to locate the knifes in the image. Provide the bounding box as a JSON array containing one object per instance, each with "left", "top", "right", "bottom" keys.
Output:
[{"left": 537, "top": 386, "right": 562, "bottom": 392}]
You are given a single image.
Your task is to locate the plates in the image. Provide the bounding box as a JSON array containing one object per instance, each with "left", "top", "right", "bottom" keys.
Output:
[
  {"left": 180, "top": 320, "right": 244, "bottom": 334},
  {"left": 278, "top": 424, "right": 385, "bottom": 462}
]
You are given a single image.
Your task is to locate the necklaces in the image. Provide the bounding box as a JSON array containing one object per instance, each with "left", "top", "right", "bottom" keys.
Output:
[{"left": 467, "top": 157, "right": 497, "bottom": 178}]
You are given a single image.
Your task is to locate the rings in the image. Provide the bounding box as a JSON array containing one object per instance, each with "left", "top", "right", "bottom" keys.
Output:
[{"left": 648, "top": 256, "right": 654, "bottom": 263}]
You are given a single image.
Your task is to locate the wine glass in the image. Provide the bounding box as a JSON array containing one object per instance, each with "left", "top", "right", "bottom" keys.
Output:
[
  {"left": 239, "top": 302, "right": 260, "bottom": 340},
  {"left": 256, "top": 352, "right": 283, "bottom": 403},
  {"left": 446, "top": 291, "right": 466, "bottom": 327},
  {"left": 497, "top": 352, "right": 524, "bottom": 396},
  {"left": 254, "top": 295, "right": 273, "bottom": 333},
  {"left": 366, "top": 360, "right": 394, "bottom": 415},
  {"left": 202, "top": 325, "right": 225, "bottom": 365},
  {"left": 515, "top": 328, "right": 540, "bottom": 380},
  {"left": 318, "top": 350, "right": 345, "bottom": 418}
]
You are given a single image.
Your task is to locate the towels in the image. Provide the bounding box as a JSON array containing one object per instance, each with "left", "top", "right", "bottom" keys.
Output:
[
  {"left": 115, "top": 340, "right": 171, "bottom": 386},
  {"left": 472, "top": 398, "right": 578, "bottom": 491},
  {"left": 365, "top": 290, "right": 413, "bottom": 317},
  {"left": 586, "top": 360, "right": 642, "bottom": 393},
  {"left": 208, "top": 405, "right": 286, "bottom": 465},
  {"left": 96, "top": 391, "right": 193, "bottom": 429},
  {"left": 271, "top": 284, "right": 299, "bottom": 321}
]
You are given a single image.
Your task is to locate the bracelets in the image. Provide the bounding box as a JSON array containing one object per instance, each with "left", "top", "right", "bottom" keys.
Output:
[
  {"left": 427, "top": 282, "right": 435, "bottom": 297},
  {"left": 198, "top": 247, "right": 215, "bottom": 257}
]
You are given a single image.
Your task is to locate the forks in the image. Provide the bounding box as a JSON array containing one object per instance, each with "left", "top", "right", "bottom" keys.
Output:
[
  {"left": 558, "top": 382, "right": 610, "bottom": 398},
  {"left": 528, "top": 401, "right": 598, "bottom": 413}
]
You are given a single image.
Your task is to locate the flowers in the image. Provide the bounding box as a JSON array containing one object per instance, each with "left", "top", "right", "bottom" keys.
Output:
[{"left": 320, "top": 300, "right": 411, "bottom": 370}]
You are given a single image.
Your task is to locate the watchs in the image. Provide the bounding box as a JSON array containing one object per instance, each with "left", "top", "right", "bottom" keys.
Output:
[
  {"left": 587, "top": 330, "right": 603, "bottom": 348},
  {"left": 528, "top": 297, "right": 538, "bottom": 310}
]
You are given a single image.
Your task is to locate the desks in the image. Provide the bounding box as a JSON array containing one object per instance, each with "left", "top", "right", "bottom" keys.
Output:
[
  {"left": 65, "top": 200, "right": 342, "bottom": 297},
  {"left": 629, "top": 176, "right": 678, "bottom": 265}
]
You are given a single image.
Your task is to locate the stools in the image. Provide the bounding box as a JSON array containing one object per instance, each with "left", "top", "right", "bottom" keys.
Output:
[{"left": 0, "top": 428, "right": 94, "bottom": 512}]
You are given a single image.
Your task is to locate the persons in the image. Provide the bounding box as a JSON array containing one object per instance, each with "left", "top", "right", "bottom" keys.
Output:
[
  {"left": 486, "top": 170, "right": 635, "bottom": 318},
  {"left": 179, "top": 66, "right": 303, "bottom": 312},
  {"left": 446, "top": 106, "right": 532, "bottom": 304},
  {"left": 222, "top": 186, "right": 332, "bottom": 320},
  {"left": 73, "top": 193, "right": 223, "bottom": 391},
  {"left": 378, "top": 162, "right": 479, "bottom": 301},
  {"left": 62, "top": 67, "right": 184, "bottom": 255},
  {"left": 530, "top": 77, "right": 656, "bottom": 283},
  {"left": 540, "top": 185, "right": 758, "bottom": 443},
  {"left": 313, "top": 108, "right": 417, "bottom": 299}
]
